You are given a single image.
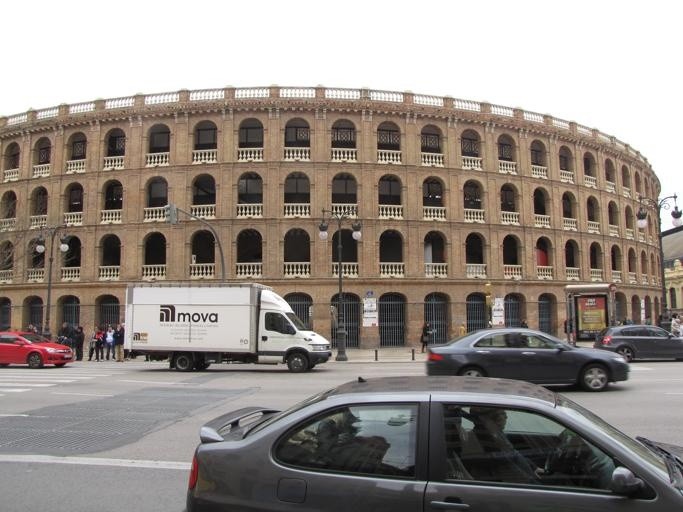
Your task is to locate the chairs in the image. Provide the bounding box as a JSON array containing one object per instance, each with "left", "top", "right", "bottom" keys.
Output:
[{"left": 443, "top": 417, "right": 476, "bottom": 480}]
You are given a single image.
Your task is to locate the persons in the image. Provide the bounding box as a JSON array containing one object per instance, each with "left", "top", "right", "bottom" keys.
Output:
[
  {"left": 610, "top": 314, "right": 662, "bottom": 327},
  {"left": 467, "top": 407, "right": 545, "bottom": 483},
  {"left": 459, "top": 321, "right": 466, "bottom": 336},
  {"left": 564, "top": 317, "right": 573, "bottom": 343},
  {"left": 521, "top": 318, "right": 528, "bottom": 328},
  {"left": 520, "top": 336, "right": 529, "bottom": 347},
  {"left": 420, "top": 321, "right": 431, "bottom": 353},
  {"left": 25, "top": 321, "right": 124, "bottom": 362},
  {"left": 670, "top": 312, "right": 681, "bottom": 337}
]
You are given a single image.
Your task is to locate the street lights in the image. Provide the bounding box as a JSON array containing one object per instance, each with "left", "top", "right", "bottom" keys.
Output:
[
  {"left": 318, "top": 207, "right": 363, "bottom": 362},
  {"left": 636, "top": 190, "right": 682, "bottom": 322},
  {"left": 35, "top": 224, "right": 72, "bottom": 341}
]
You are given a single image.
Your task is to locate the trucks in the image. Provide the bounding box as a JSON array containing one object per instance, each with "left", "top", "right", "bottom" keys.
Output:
[{"left": 120, "top": 281, "right": 336, "bottom": 375}]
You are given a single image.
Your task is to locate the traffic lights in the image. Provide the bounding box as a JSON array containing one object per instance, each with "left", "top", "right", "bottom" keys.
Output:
[{"left": 164, "top": 201, "right": 176, "bottom": 226}]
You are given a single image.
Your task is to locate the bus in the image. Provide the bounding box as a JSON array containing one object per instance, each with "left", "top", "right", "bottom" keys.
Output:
[{"left": 0, "top": 330, "right": 76, "bottom": 370}]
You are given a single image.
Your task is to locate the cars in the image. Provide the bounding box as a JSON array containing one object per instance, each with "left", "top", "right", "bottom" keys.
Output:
[
  {"left": 416, "top": 326, "right": 631, "bottom": 393},
  {"left": 176, "top": 371, "right": 682, "bottom": 512},
  {"left": 590, "top": 324, "right": 682, "bottom": 363}
]
